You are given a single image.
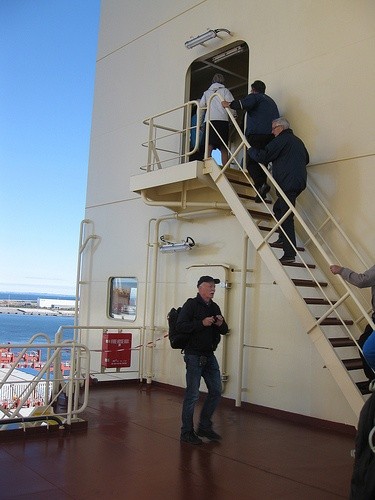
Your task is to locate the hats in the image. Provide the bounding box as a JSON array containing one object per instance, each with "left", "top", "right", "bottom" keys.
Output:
[{"left": 197, "top": 276, "right": 220, "bottom": 287}]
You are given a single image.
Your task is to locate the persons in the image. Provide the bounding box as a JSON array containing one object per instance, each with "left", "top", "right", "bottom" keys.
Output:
[
  {"left": 175, "top": 276, "right": 228, "bottom": 444},
  {"left": 199, "top": 73, "right": 238, "bottom": 167},
  {"left": 189, "top": 99, "right": 206, "bottom": 162},
  {"left": 222, "top": 80, "right": 280, "bottom": 203},
  {"left": 329, "top": 265, "right": 375, "bottom": 500},
  {"left": 246, "top": 119, "right": 309, "bottom": 264}
]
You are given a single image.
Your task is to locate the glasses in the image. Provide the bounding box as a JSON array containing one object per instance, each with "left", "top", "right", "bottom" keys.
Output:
[{"left": 272, "top": 125, "right": 282, "bottom": 131}]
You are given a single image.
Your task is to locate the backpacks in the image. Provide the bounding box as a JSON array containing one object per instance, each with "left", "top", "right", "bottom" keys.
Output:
[{"left": 167, "top": 298, "right": 200, "bottom": 354}]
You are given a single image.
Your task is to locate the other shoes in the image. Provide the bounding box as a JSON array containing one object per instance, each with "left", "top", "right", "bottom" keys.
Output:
[
  {"left": 358, "top": 382, "right": 368, "bottom": 388},
  {"left": 255, "top": 182, "right": 271, "bottom": 203}
]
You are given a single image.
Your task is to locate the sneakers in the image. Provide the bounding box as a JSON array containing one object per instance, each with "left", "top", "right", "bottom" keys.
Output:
[
  {"left": 269, "top": 239, "right": 284, "bottom": 248},
  {"left": 279, "top": 256, "right": 295, "bottom": 263},
  {"left": 180, "top": 430, "right": 202, "bottom": 445},
  {"left": 196, "top": 421, "right": 222, "bottom": 441}
]
